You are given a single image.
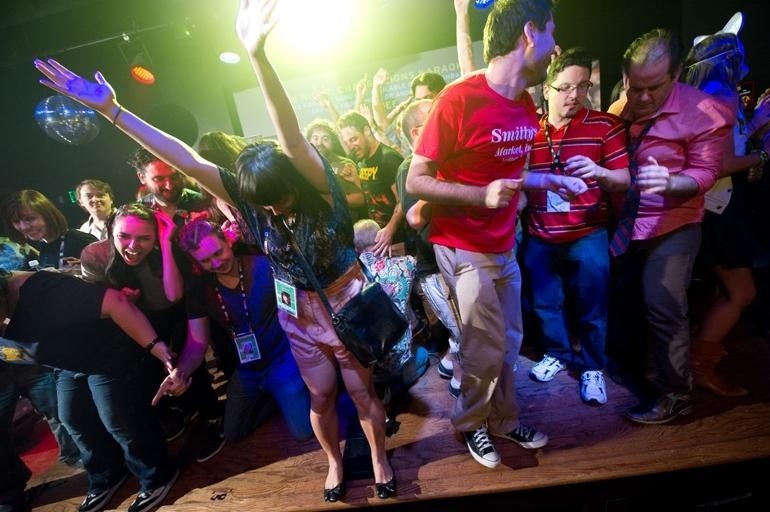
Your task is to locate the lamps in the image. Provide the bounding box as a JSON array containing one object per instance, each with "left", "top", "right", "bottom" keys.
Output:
[{"left": 16, "top": 22, "right": 244, "bottom": 88}]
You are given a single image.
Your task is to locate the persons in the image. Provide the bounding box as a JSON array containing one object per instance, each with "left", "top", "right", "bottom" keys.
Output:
[{"left": 406, "top": 0, "right": 588, "bottom": 469}]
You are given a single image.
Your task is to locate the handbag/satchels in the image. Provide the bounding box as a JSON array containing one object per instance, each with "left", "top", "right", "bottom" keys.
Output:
[{"left": 328, "top": 283, "right": 410, "bottom": 370}]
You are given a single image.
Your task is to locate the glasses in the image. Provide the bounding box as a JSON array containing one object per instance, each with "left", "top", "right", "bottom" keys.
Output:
[{"left": 546, "top": 80, "right": 594, "bottom": 94}]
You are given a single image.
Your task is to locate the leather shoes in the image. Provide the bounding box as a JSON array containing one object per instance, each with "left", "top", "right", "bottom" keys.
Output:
[
  {"left": 373, "top": 479, "right": 396, "bottom": 498},
  {"left": 324, "top": 481, "right": 345, "bottom": 503},
  {"left": 624, "top": 393, "right": 695, "bottom": 426}
]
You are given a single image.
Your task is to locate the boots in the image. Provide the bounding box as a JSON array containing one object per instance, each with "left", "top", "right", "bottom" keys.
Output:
[{"left": 688, "top": 341, "right": 747, "bottom": 398}]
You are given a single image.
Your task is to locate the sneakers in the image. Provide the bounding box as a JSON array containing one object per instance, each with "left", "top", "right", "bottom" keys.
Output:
[
  {"left": 163, "top": 405, "right": 199, "bottom": 441},
  {"left": 461, "top": 419, "right": 500, "bottom": 470},
  {"left": 438, "top": 354, "right": 462, "bottom": 399},
  {"left": 489, "top": 423, "right": 549, "bottom": 451},
  {"left": 123, "top": 471, "right": 181, "bottom": 511},
  {"left": 579, "top": 371, "right": 609, "bottom": 406},
  {"left": 195, "top": 419, "right": 227, "bottom": 463},
  {"left": 77, "top": 471, "right": 127, "bottom": 511},
  {"left": 528, "top": 354, "right": 570, "bottom": 382}
]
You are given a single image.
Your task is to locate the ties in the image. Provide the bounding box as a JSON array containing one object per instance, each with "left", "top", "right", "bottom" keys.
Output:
[{"left": 607, "top": 117, "right": 658, "bottom": 259}]
